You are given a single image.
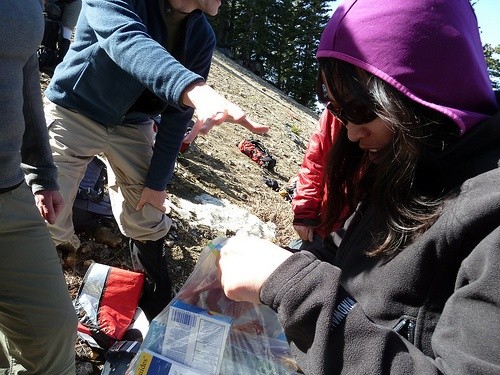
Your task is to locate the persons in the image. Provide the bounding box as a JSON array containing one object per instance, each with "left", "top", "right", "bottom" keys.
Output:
[
  {"left": 0, "top": 0, "right": 269, "bottom": 375},
  {"left": 216, "top": 0, "right": 500, "bottom": 375},
  {"left": 286, "top": 99, "right": 372, "bottom": 253}
]
